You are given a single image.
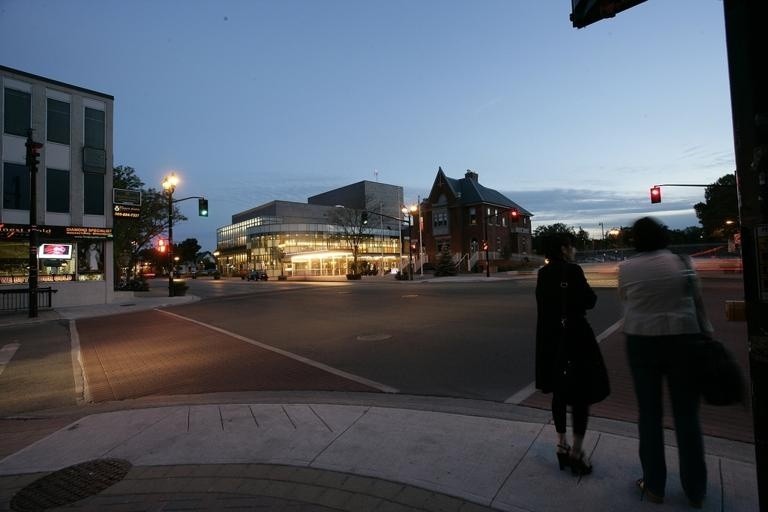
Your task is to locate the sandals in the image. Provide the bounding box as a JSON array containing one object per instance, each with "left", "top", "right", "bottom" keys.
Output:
[{"left": 637, "top": 479, "right": 663, "bottom": 505}]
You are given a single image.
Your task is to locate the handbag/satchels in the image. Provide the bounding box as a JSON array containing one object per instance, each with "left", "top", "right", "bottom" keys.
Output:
[{"left": 699, "top": 340, "right": 746, "bottom": 408}]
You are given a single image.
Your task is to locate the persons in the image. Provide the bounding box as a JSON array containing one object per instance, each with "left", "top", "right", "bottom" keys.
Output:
[
  {"left": 616, "top": 216, "right": 716, "bottom": 509},
  {"left": 84, "top": 240, "right": 101, "bottom": 270},
  {"left": 535, "top": 233, "right": 611, "bottom": 476}
]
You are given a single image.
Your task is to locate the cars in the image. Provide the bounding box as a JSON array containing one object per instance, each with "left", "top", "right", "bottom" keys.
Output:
[
  {"left": 142, "top": 271, "right": 157, "bottom": 279},
  {"left": 172, "top": 267, "right": 218, "bottom": 276},
  {"left": 246, "top": 270, "right": 268, "bottom": 281},
  {"left": 579, "top": 253, "right": 627, "bottom": 261}
]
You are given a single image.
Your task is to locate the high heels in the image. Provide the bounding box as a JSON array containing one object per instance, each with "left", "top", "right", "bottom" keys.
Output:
[
  {"left": 570, "top": 447, "right": 592, "bottom": 477},
  {"left": 556, "top": 445, "right": 572, "bottom": 471}
]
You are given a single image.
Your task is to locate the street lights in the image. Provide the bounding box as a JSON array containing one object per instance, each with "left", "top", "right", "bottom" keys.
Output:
[
  {"left": 400, "top": 205, "right": 418, "bottom": 281},
  {"left": 160, "top": 173, "right": 175, "bottom": 287},
  {"left": 610, "top": 227, "right": 625, "bottom": 262}
]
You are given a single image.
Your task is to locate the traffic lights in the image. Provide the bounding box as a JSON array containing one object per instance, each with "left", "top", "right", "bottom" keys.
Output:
[
  {"left": 511, "top": 210, "right": 520, "bottom": 220},
  {"left": 651, "top": 188, "right": 660, "bottom": 202},
  {"left": 483, "top": 243, "right": 488, "bottom": 250},
  {"left": 199, "top": 199, "right": 207, "bottom": 216},
  {"left": 361, "top": 213, "right": 369, "bottom": 224},
  {"left": 158, "top": 238, "right": 165, "bottom": 252}
]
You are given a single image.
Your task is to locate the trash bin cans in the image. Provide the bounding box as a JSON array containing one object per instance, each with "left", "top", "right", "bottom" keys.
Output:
[
  {"left": 192, "top": 273, "right": 196, "bottom": 279},
  {"left": 478, "top": 264, "right": 483, "bottom": 273},
  {"left": 173, "top": 284, "right": 188, "bottom": 296}
]
